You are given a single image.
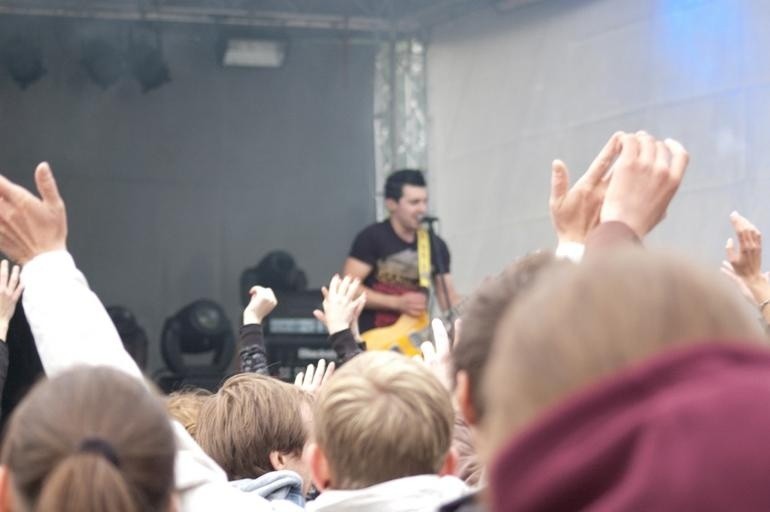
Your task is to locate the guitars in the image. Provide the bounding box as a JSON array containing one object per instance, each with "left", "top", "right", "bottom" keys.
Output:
[{"left": 360, "top": 294, "right": 471, "bottom": 360}]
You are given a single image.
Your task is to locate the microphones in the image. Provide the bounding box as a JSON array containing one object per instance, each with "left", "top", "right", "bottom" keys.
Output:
[{"left": 415, "top": 214, "right": 439, "bottom": 223}]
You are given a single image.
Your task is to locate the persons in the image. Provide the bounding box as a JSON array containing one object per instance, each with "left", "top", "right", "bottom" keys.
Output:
[{"left": 0, "top": 129, "right": 767, "bottom": 510}]
